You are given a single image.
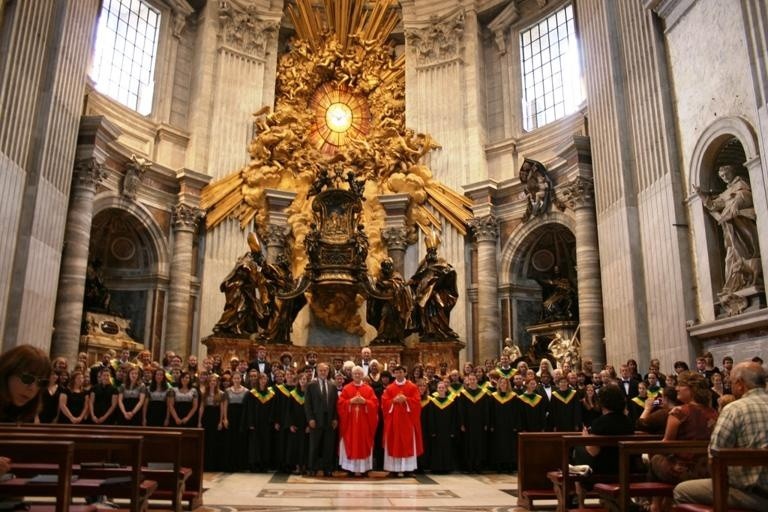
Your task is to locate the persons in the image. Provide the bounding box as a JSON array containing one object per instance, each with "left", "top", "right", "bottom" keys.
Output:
[
  {"left": 503, "top": 337, "right": 523, "bottom": 362},
  {"left": 252, "top": 248, "right": 312, "bottom": 344},
  {"left": 303, "top": 222, "right": 321, "bottom": 269},
  {"left": 210, "top": 251, "right": 264, "bottom": 340},
  {"left": 252, "top": 24, "right": 439, "bottom": 206},
  {"left": 362, "top": 254, "right": 414, "bottom": 346},
  {"left": 690, "top": 165, "right": 762, "bottom": 318},
  {"left": 355, "top": 223, "right": 370, "bottom": 264},
  {"left": 547, "top": 331, "right": 578, "bottom": 374},
  {"left": 539, "top": 264, "right": 580, "bottom": 324},
  {"left": 2, "top": 348, "right": 767, "bottom": 512},
  {"left": 405, "top": 233, "right": 464, "bottom": 342}
]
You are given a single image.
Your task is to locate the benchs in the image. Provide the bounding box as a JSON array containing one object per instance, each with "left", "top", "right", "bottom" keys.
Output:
[
  {"left": 517, "top": 432, "right": 768, "bottom": 512},
  {"left": 0, "top": 423, "right": 205, "bottom": 512}
]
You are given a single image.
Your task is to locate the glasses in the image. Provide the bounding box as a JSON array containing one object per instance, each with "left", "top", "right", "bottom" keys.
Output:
[{"left": 17, "top": 372, "right": 50, "bottom": 388}]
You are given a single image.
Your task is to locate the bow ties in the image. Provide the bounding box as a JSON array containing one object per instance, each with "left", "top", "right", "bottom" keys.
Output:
[
  {"left": 545, "top": 386, "right": 550, "bottom": 388},
  {"left": 259, "top": 361, "right": 264, "bottom": 363},
  {"left": 622, "top": 381, "right": 629, "bottom": 383}
]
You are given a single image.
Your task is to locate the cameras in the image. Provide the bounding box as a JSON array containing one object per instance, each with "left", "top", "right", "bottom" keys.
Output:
[{"left": 653, "top": 399, "right": 662, "bottom": 404}]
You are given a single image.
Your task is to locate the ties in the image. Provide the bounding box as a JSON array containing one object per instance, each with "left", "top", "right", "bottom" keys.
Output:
[{"left": 322, "top": 380, "right": 326, "bottom": 404}]
